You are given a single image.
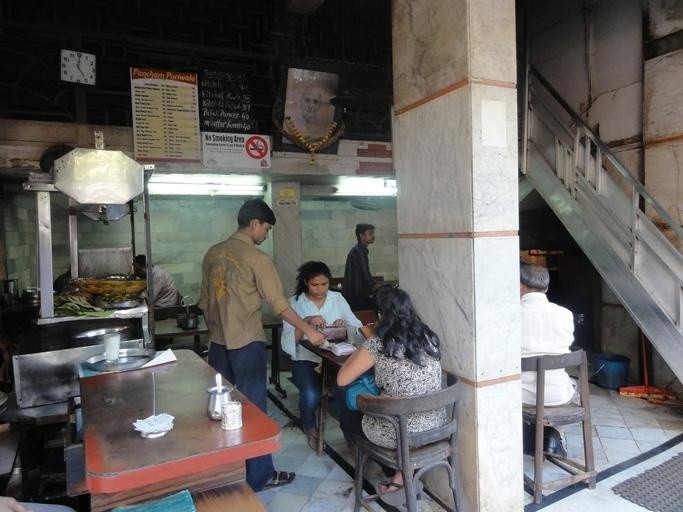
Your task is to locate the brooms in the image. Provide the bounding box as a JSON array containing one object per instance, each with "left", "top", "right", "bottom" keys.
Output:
[{"left": 619, "top": 327, "right": 677, "bottom": 402}]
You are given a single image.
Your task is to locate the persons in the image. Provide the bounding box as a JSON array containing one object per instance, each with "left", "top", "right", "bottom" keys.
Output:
[
  {"left": 342, "top": 223, "right": 376, "bottom": 311},
  {"left": 520, "top": 263, "right": 580, "bottom": 457},
  {"left": 280, "top": 261, "right": 366, "bottom": 450},
  {"left": 335, "top": 286, "right": 447, "bottom": 493},
  {"left": 196, "top": 198, "right": 324, "bottom": 492},
  {"left": 132, "top": 255, "right": 176, "bottom": 320}
]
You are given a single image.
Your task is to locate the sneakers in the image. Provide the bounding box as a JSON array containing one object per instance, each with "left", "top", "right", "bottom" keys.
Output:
[{"left": 302, "top": 424, "right": 326, "bottom": 450}]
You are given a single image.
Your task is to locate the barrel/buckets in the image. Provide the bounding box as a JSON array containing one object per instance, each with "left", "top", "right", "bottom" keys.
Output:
[{"left": 594, "top": 354, "right": 630, "bottom": 388}]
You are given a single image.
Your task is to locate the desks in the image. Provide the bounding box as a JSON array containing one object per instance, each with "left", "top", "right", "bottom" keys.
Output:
[
  {"left": 76, "top": 347, "right": 283, "bottom": 511},
  {"left": 300, "top": 338, "right": 357, "bottom": 457},
  {"left": 153, "top": 312, "right": 287, "bottom": 397}
]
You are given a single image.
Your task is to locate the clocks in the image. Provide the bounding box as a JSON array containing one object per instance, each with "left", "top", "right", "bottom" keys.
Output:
[{"left": 57, "top": 49, "right": 98, "bottom": 89}]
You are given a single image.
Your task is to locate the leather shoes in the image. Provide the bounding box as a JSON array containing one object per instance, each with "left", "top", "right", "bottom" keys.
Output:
[{"left": 524, "top": 422, "right": 567, "bottom": 461}]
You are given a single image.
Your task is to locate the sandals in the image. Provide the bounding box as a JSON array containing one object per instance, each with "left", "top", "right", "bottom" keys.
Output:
[
  {"left": 378, "top": 480, "right": 403, "bottom": 494},
  {"left": 254, "top": 471, "right": 295, "bottom": 492}
]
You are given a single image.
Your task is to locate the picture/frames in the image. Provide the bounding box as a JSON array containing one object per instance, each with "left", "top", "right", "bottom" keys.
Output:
[{"left": 273, "top": 58, "right": 345, "bottom": 154}]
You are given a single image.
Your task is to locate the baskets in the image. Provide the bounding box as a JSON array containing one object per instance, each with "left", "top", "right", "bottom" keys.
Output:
[{"left": 316, "top": 325, "right": 347, "bottom": 340}]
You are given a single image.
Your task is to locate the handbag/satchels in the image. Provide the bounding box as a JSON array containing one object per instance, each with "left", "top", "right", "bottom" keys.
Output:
[{"left": 345, "top": 374, "right": 381, "bottom": 411}]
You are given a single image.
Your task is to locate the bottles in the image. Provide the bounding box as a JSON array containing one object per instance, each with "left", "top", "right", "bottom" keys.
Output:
[{"left": 206, "top": 385, "right": 235, "bottom": 421}]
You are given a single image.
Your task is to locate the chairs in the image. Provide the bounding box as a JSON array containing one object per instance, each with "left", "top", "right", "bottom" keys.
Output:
[
  {"left": 354, "top": 371, "right": 466, "bottom": 512},
  {"left": 522, "top": 351, "right": 597, "bottom": 505}
]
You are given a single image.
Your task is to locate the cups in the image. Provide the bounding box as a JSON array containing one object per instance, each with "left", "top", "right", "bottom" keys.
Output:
[
  {"left": 102, "top": 331, "right": 121, "bottom": 363},
  {"left": 346, "top": 325, "right": 357, "bottom": 346}
]
